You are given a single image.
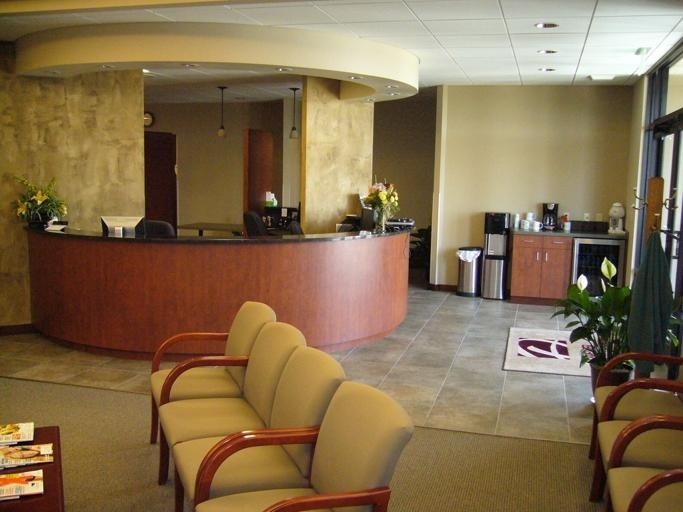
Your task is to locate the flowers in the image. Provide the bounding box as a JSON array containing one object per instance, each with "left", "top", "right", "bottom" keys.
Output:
[
  {"left": 11, "top": 173, "right": 68, "bottom": 221},
  {"left": 551, "top": 256, "right": 680, "bottom": 370},
  {"left": 363, "top": 175, "right": 400, "bottom": 234}
]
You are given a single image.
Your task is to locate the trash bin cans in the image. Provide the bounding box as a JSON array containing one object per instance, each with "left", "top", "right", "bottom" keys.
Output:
[{"left": 456, "top": 246, "right": 481, "bottom": 296}]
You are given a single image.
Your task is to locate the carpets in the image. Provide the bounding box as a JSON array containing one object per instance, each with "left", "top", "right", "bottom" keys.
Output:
[
  {"left": 0, "top": 375, "right": 605, "bottom": 512},
  {"left": 503, "top": 325, "right": 595, "bottom": 377}
]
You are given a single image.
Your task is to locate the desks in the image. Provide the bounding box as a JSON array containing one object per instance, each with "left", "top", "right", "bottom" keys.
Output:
[{"left": 0, "top": 426, "right": 65, "bottom": 511}]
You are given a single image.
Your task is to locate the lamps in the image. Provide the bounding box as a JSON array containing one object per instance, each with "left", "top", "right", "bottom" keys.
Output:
[
  {"left": 217, "top": 87, "right": 228, "bottom": 136},
  {"left": 289, "top": 87, "right": 299, "bottom": 139}
]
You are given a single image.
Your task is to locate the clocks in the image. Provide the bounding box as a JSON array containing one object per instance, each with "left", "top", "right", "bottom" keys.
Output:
[{"left": 144, "top": 111, "right": 155, "bottom": 127}]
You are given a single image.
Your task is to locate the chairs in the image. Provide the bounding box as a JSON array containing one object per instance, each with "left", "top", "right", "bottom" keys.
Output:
[
  {"left": 290, "top": 220, "right": 304, "bottom": 234},
  {"left": 143, "top": 220, "right": 174, "bottom": 238},
  {"left": 589, "top": 350, "right": 682, "bottom": 511},
  {"left": 244, "top": 211, "right": 269, "bottom": 237}
]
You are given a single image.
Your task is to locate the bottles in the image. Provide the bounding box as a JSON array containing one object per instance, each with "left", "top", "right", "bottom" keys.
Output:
[{"left": 514, "top": 214, "right": 519, "bottom": 231}]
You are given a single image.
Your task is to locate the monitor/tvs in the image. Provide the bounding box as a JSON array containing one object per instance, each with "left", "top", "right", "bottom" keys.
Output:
[{"left": 100, "top": 215, "right": 145, "bottom": 238}]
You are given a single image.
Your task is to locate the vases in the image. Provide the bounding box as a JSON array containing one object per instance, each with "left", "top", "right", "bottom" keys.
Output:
[
  {"left": 375, "top": 208, "right": 385, "bottom": 236},
  {"left": 586, "top": 357, "right": 633, "bottom": 398},
  {"left": 26, "top": 215, "right": 49, "bottom": 229}
]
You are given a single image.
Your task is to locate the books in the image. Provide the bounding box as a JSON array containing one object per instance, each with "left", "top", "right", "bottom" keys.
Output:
[{"left": 0, "top": 422, "right": 54, "bottom": 501}]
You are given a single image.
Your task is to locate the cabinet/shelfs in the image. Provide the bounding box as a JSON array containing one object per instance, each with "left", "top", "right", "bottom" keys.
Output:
[{"left": 510, "top": 231, "right": 573, "bottom": 307}]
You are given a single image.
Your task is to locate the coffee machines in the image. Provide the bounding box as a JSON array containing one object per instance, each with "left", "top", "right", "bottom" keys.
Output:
[
  {"left": 541, "top": 202, "right": 558, "bottom": 230},
  {"left": 608, "top": 202, "right": 624, "bottom": 233}
]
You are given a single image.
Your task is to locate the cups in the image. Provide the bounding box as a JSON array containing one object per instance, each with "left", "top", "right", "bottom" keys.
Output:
[
  {"left": 561, "top": 222, "right": 571, "bottom": 233},
  {"left": 521, "top": 212, "right": 543, "bottom": 232}
]
